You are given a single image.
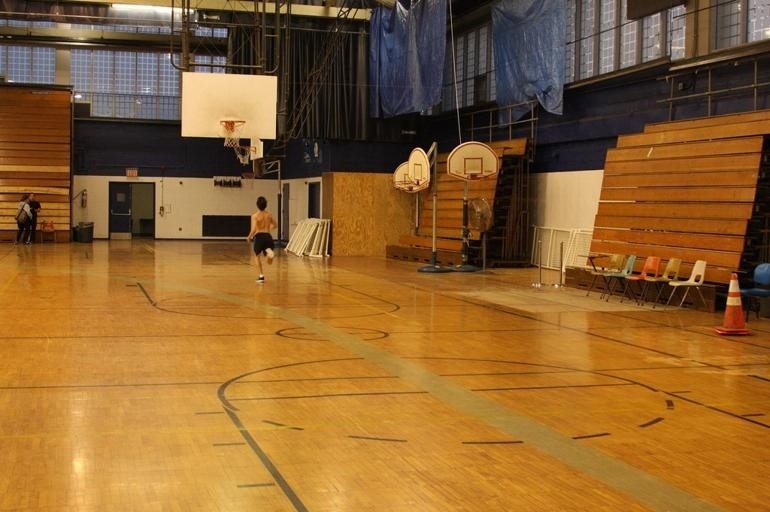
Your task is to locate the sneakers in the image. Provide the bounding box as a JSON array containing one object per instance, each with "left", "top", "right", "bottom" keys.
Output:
[{"left": 256, "top": 276, "right": 264, "bottom": 282}]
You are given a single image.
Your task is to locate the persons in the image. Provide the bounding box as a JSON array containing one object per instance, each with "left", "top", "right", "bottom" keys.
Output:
[
  {"left": 247, "top": 195, "right": 278, "bottom": 284},
  {"left": 15, "top": 195, "right": 33, "bottom": 244},
  {"left": 28, "top": 193, "right": 41, "bottom": 243}
]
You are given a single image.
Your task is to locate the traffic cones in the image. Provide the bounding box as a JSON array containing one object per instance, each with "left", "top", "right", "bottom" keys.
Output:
[{"left": 714, "top": 272, "right": 754, "bottom": 337}]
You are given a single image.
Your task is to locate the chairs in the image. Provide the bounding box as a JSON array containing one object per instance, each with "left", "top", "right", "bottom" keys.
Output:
[
  {"left": 739, "top": 261, "right": 770, "bottom": 319},
  {"left": 619, "top": 255, "right": 664, "bottom": 305},
  {"left": 39, "top": 221, "right": 58, "bottom": 242},
  {"left": 665, "top": 258, "right": 709, "bottom": 309},
  {"left": 639, "top": 257, "right": 683, "bottom": 309},
  {"left": 600, "top": 253, "right": 638, "bottom": 303}
]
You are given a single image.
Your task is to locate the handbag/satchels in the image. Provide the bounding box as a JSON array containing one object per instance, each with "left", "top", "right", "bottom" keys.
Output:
[{"left": 15, "top": 209, "right": 30, "bottom": 226}]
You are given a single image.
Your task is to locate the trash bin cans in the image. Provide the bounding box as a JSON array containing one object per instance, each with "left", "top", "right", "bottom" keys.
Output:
[
  {"left": 753, "top": 263, "right": 769, "bottom": 318},
  {"left": 72, "top": 222, "right": 94, "bottom": 243}
]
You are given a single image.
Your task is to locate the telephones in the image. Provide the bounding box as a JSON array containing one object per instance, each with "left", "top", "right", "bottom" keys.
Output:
[{"left": 83, "top": 191, "right": 87, "bottom": 208}]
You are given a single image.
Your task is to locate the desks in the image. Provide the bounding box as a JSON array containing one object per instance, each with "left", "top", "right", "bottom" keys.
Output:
[{"left": 577, "top": 249, "right": 617, "bottom": 300}]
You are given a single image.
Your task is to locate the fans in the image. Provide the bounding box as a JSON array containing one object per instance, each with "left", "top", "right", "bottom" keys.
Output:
[{"left": 469, "top": 195, "right": 497, "bottom": 275}]
